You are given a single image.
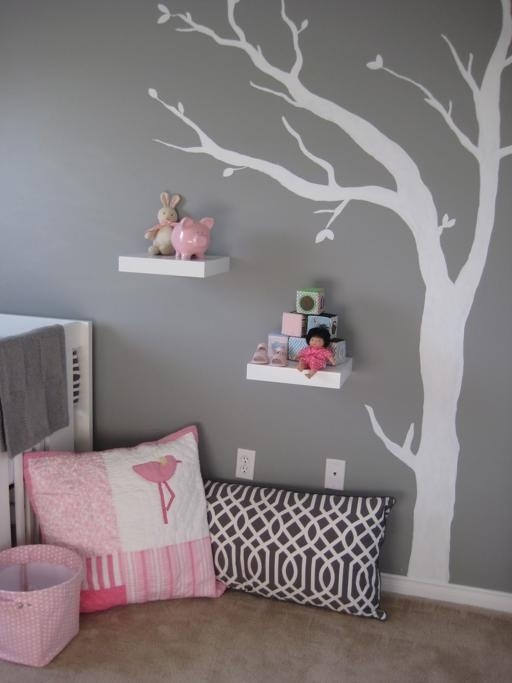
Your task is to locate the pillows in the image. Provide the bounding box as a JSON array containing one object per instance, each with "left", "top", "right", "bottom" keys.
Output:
[
  {"left": 202, "top": 476, "right": 397, "bottom": 622},
  {"left": 20, "top": 423, "right": 226, "bottom": 615}
]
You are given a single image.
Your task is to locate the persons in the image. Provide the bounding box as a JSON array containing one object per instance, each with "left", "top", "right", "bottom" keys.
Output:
[{"left": 292, "top": 327, "right": 335, "bottom": 378}]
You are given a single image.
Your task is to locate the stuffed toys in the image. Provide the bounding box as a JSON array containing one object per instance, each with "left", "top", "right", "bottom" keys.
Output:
[{"left": 143, "top": 191, "right": 182, "bottom": 256}]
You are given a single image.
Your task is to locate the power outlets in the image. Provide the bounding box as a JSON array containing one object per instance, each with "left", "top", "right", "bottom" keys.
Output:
[{"left": 233, "top": 447, "right": 257, "bottom": 481}]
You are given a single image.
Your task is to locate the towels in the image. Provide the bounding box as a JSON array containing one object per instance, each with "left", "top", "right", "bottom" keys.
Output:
[{"left": 1, "top": 322, "right": 71, "bottom": 459}]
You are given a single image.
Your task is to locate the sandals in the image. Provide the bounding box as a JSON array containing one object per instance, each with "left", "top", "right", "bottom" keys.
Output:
[{"left": 252, "top": 342, "right": 287, "bottom": 367}]
